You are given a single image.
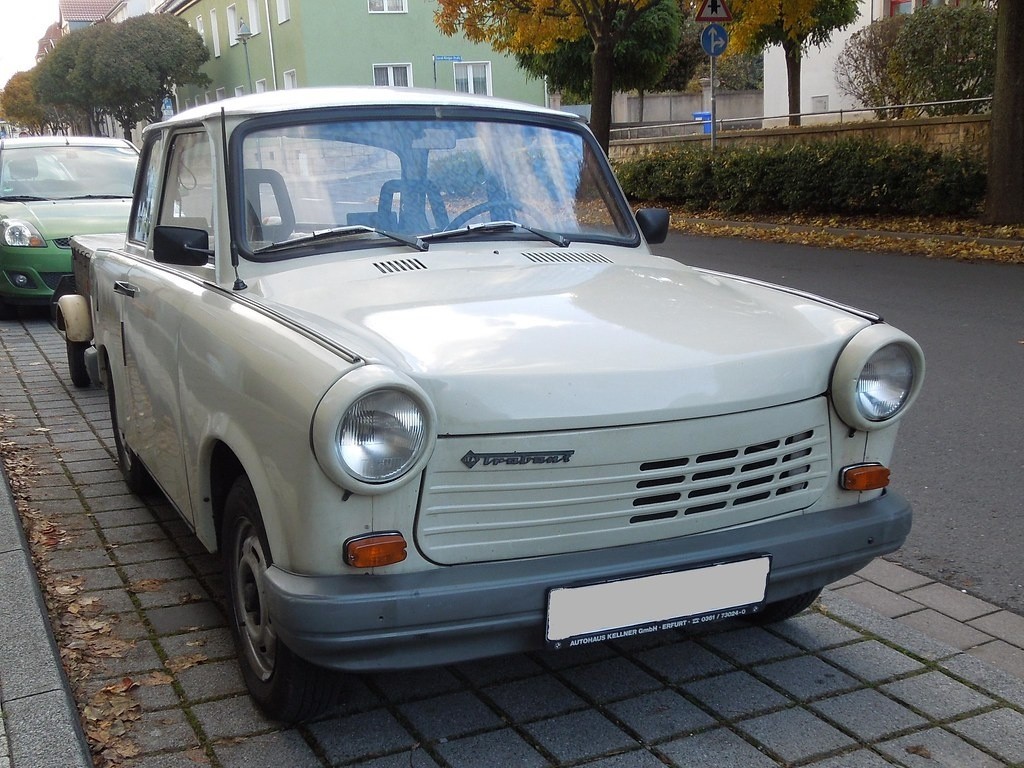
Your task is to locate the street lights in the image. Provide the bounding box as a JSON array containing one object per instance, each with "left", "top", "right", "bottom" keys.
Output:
[{"left": 235, "top": 17, "right": 253, "bottom": 95}]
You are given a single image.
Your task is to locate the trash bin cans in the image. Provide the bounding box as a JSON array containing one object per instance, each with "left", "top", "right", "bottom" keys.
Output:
[{"left": 691, "top": 112, "right": 712, "bottom": 133}]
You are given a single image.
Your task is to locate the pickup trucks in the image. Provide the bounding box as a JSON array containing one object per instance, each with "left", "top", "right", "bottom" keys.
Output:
[{"left": 57, "top": 84, "right": 925, "bottom": 725}]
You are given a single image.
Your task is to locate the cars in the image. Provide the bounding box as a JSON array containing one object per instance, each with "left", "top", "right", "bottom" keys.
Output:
[
  {"left": 0, "top": 150, "right": 75, "bottom": 184},
  {"left": 0, "top": 137, "right": 189, "bottom": 306}
]
